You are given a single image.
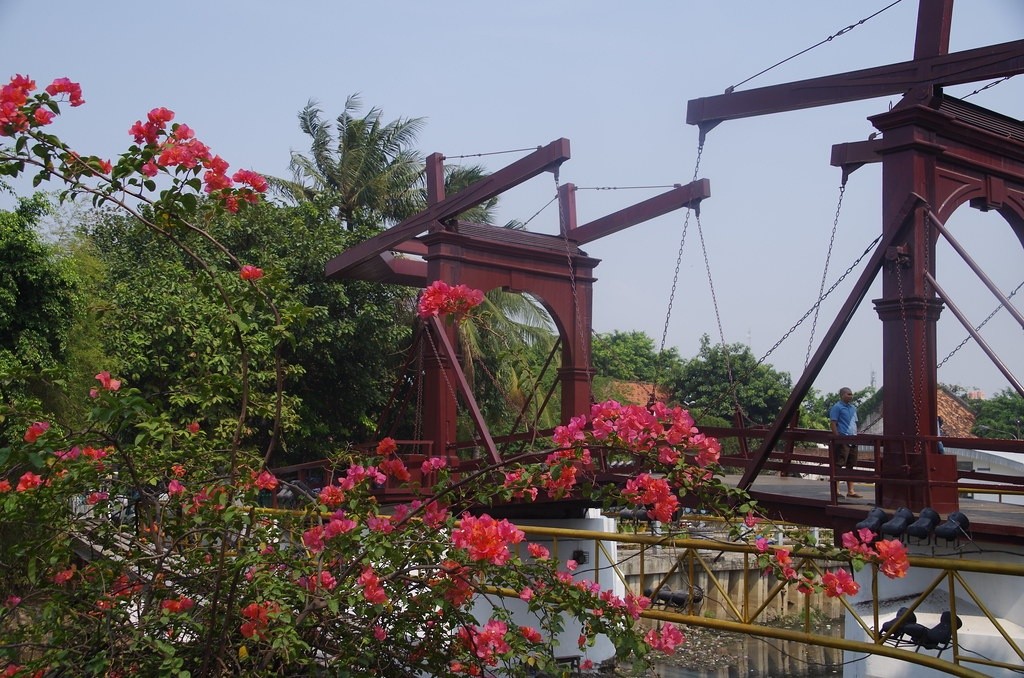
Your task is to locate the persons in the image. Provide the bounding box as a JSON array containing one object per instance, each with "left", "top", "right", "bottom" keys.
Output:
[{"left": 829, "top": 387, "right": 864, "bottom": 499}]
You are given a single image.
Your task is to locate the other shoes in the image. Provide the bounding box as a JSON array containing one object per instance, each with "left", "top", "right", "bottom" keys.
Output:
[
  {"left": 837, "top": 492, "right": 845, "bottom": 499},
  {"left": 845, "top": 493, "right": 863, "bottom": 498}
]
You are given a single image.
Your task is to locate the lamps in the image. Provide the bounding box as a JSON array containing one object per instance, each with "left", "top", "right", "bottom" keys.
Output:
[
  {"left": 906, "top": 507, "right": 941, "bottom": 546},
  {"left": 903, "top": 622, "right": 930, "bottom": 646},
  {"left": 922, "top": 611, "right": 962, "bottom": 650},
  {"left": 878, "top": 606, "right": 917, "bottom": 640},
  {"left": 934, "top": 511, "right": 969, "bottom": 547},
  {"left": 855, "top": 508, "right": 887, "bottom": 540},
  {"left": 879, "top": 507, "right": 914, "bottom": 544}
]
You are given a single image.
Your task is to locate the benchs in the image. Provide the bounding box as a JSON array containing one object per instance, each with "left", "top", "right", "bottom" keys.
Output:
[{"left": 519, "top": 654, "right": 584, "bottom": 678}]
